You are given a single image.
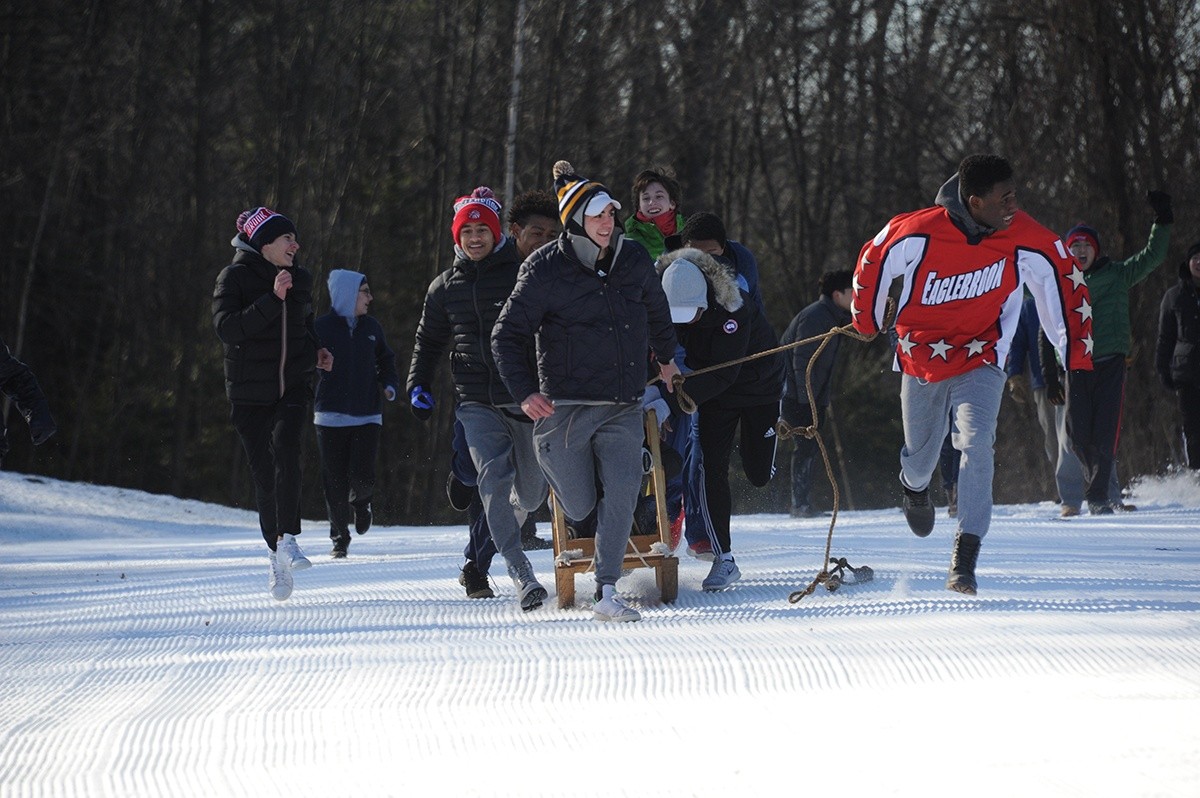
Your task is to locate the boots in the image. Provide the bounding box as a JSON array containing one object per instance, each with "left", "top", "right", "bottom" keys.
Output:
[
  {"left": 902, "top": 483, "right": 934, "bottom": 537},
  {"left": 947, "top": 534, "right": 983, "bottom": 594}
]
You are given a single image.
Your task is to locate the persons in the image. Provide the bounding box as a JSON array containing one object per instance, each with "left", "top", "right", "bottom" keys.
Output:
[
  {"left": 210, "top": 207, "right": 335, "bottom": 601},
  {"left": 322, "top": 154, "right": 1200, "bottom": 622}
]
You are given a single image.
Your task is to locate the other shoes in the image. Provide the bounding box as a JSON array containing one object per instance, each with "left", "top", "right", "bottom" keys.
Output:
[
  {"left": 1110, "top": 502, "right": 1137, "bottom": 512},
  {"left": 792, "top": 505, "right": 833, "bottom": 518},
  {"left": 1088, "top": 499, "right": 1115, "bottom": 515},
  {"left": 1061, "top": 505, "right": 1080, "bottom": 516}
]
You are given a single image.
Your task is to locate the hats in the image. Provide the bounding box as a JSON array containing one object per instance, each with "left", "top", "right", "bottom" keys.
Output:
[
  {"left": 1066, "top": 223, "right": 1099, "bottom": 255},
  {"left": 552, "top": 160, "right": 613, "bottom": 231},
  {"left": 236, "top": 207, "right": 297, "bottom": 252},
  {"left": 1187, "top": 243, "right": 1200, "bottom": 266},
  {"left": 668, "top": 306, "right": 698, "bottom": 323},
  {"left": 450, "top": 188, "right": 502, "bottom": 247},
  {"left": 582, "top": 190, "right": 623, "bottom": 217}
]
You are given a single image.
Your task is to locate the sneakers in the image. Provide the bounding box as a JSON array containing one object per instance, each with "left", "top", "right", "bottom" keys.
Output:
[
  {"left": 446, "top": 469, "right": 473, "bottom": 511},
  {"left": 509, "top": 493, "right": 519, "bottom": 509},
  {"left": 330, "top": 539, "right": 347, "bottom": 560},
  {"left": 267, "top": 548, "right": 293, "bottom": 601},
  {"left": 275, "top": 534, "right": 312, "bottom": 572},
  {"left": 687, "top": 541, "right": 716, "bottom": 562},
  {"left": 701, "top": 554, "right": 741, "bottom": 593},
  {"left": 459, "top": 559, "right": 494, "bottom": 599},
  {"left": 509, "top": 564, "right": 547, "bottom": 612},
  {"left": 354, "top": 503, "right": 372, "bottom": 535},
  {"left": 667, "top": 506, "right": 685, "bottom": 557},
  {"left": 591, "top": 583, "right": 642, "bottom": 621}
]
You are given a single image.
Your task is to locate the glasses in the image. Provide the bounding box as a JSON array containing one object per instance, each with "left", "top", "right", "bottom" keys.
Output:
[{"left": 359, "top": 288, "right": 370, "bottom": 295}]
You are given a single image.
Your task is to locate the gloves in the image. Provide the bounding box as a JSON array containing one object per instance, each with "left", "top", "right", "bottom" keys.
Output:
[
  {"left": 1043, "top": 374, "right": 1066, "bottom": 404},
  {"left": 409, "top": 385, "right": 434, "bottom": 421},
  {"left": 1146, "top": 190, "right": 1174, "bottom": 224}
]
